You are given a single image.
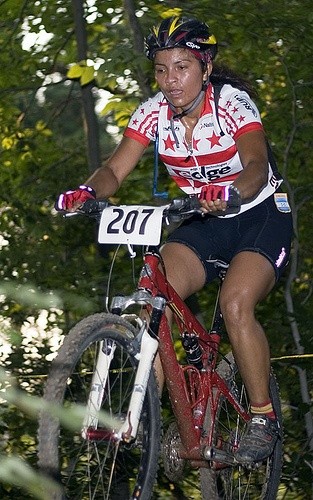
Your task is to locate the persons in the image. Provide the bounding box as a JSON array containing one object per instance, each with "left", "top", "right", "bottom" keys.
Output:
[{"left": 57, "top": 15, "right": 293, "bottom": 463}]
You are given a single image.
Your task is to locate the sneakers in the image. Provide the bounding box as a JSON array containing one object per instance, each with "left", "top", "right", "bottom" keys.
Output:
[
  {"left": 113, "top": 412, "right": 143, "bottom": 452},
  {"left": 234, "top": 415, "right": 280, "bottom": 461}
]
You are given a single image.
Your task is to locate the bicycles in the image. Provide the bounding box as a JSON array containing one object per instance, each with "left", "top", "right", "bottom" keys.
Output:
[{"left": 36, "top": 188, "right": 284, "bottom": 500}]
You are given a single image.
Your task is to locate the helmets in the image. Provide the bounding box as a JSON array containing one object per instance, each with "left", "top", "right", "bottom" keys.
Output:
[{"left": 144, "top": 16, "right": 217, "bottom": 64}]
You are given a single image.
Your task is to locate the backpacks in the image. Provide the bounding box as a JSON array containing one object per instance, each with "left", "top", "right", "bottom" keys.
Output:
[{"left": 209, "top": 69, "right": 259, "bottom": 134}]
